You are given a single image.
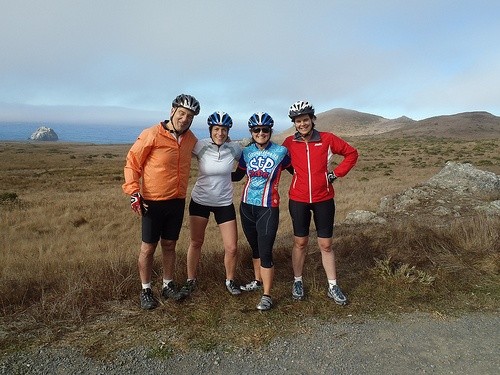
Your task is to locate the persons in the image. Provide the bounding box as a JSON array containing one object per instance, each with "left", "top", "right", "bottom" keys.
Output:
[
  {"left": 231, "top": 112, "right": 295, "bottom": 311},
  {"left": 121, "top": 94, "right": 200, "bottom": 311},
  {"left": 280, "top": 100, "right": 358, "bottom": 305},
  {"left": 180, "top": 111, "right": 243, "bottom": 296}
]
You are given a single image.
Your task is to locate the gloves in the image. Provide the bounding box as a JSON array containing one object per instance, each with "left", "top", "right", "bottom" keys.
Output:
[
  {"left": 328, "top": 171, "right": 336, "bottom": 183},
  {"left": 131, "top": 192, "right": 148, "bottom": 216}
]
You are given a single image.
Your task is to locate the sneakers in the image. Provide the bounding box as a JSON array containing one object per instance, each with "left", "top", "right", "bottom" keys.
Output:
[
  {"left": 180, "top": 282, "right": 194, "bottom": 295},
  {"left": 162, "top": 281, "right": 184, "bottom": 300},
  {"left": 327, "top": 284, "right": 347, "bottom": 305},
  {"left": 291, "top": 280, "right": 304, "bottom": 300},
  {"left": 225, "top": 280, "right": 241, "bottom": 295},
  {"left": 240, "top": 281, "right": 262, "bottom": 291},
  {"left": 140, "top": 288, "right": 156, "bottom": 309},
  {"left": 256, "top": 296, "right": 272, "bottom": 310}
]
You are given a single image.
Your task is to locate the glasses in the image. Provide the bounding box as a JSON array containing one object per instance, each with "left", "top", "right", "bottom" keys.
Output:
[{"left": 252, "top": 128, "right": 271, "bottom": 134}]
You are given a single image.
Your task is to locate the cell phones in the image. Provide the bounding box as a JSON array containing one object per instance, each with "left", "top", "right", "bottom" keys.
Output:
[{"left": 141, "top": 206, "right": 148, "bottom": 216}]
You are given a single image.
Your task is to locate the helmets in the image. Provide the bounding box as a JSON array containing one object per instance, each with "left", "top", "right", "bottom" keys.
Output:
[
  {"left": 208, "top": 112, "right": 232, "bottom": 128},
  {"left": 289, "top": 101, "right": 315, "bottom": 117},
  {"left": 248, "top": 111, "right": 274, "bottom": 127},
  {"left": 171, "top": 94, "right": 200, "bottom": 114}
]
